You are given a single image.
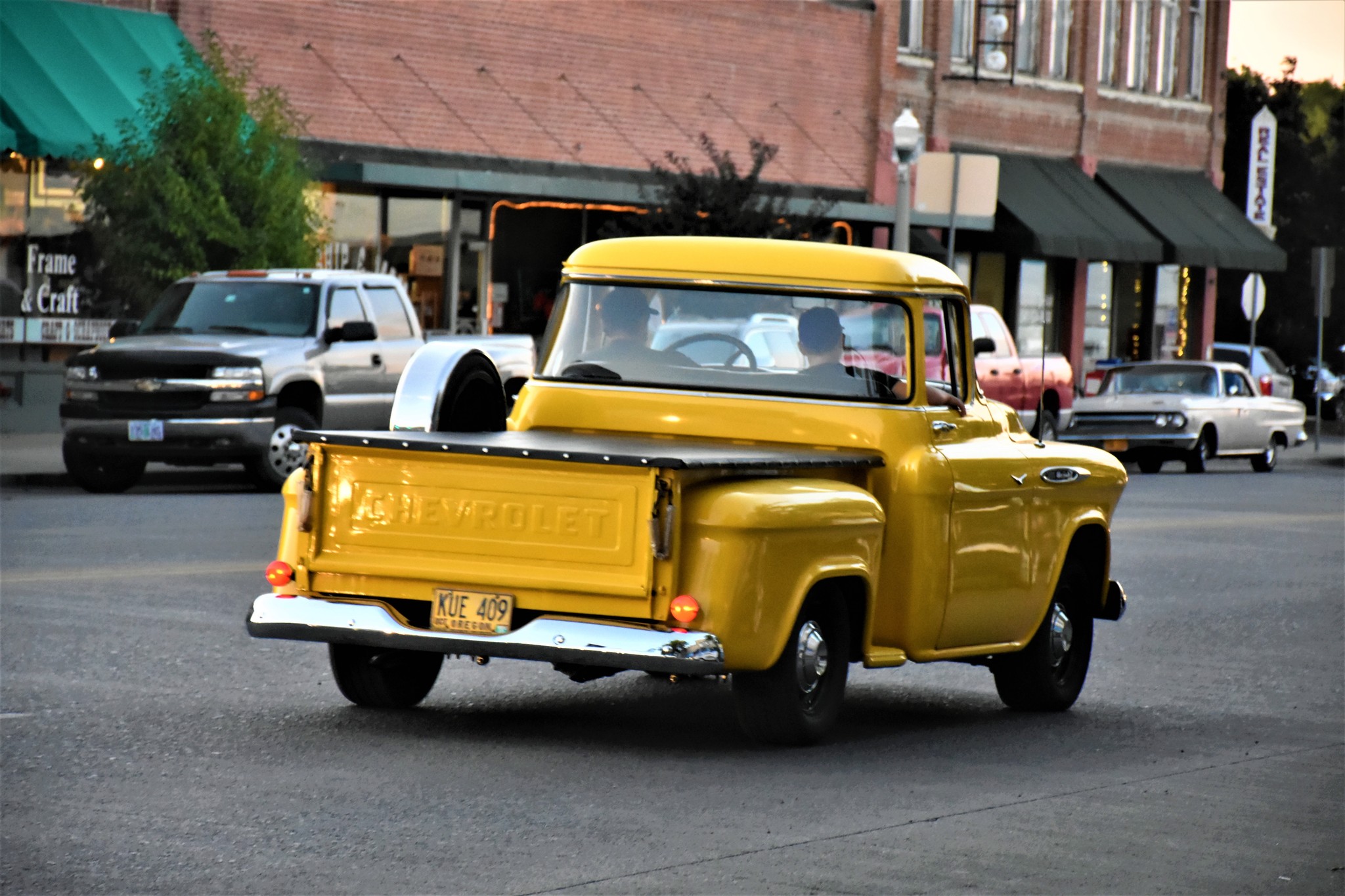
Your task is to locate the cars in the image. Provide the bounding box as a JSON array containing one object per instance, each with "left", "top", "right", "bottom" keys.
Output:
[
  {"left": 773, "top": 303, "right": 1074, "bottom": 450},
  {"left": 1070, "top": 358, "right": 1308, "bottom": 474},
  {"left": 240, "top": 236, "right": 1126, "bottom": 717}
]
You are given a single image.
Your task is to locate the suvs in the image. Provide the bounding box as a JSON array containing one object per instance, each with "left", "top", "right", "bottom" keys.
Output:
[
  {"left": 1209, "top": 343, "right": 1295, "bottom": 399},
  {"left": 657, "top": 313, "right": 805, "bottom": 375}
]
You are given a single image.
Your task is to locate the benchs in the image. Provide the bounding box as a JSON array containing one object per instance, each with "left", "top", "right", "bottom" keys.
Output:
[{"left": 564, "top": 358, "right": 898, "bottom": 403}]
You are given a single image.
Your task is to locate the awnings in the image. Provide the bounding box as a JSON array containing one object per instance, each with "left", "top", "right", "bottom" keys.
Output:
[
  {"left": 950, "top": 142, "right": 1287, "bottom": 272},
  {"left": 0, "top": 0, "right": 279, "bottom": 175}
]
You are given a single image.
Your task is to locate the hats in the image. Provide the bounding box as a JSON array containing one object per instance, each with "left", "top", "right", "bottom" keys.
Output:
[
  {"left": 600, "top": 287, "right": 660, "bottom": 325},
  {"left": 798, "top": 306, "right": 845, "bottom": 345}
]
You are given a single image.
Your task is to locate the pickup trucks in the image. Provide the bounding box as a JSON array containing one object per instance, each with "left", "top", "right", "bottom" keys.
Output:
[{"left": 58, "top": 268, "right": 535, "bottom": 495}]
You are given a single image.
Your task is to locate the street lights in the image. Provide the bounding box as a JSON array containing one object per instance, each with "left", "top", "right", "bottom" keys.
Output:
[{"left": 890, "top": 103, "right": 923, "bottom": 256}]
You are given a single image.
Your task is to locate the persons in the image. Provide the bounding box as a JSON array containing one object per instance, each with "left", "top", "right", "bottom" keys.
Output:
[
  {"left": 573, "top": 287, "right": 701, "bottom": 368},
  {"left": 797, "top": 306, "right": 966, "bottom": 417}
]
